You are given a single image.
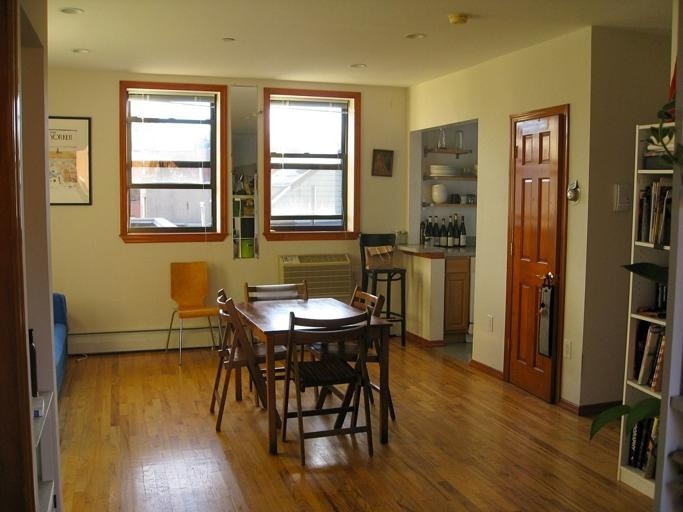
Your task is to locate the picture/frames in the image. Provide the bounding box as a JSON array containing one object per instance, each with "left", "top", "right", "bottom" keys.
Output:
[
  {"left": 48, "top": 115, "right": 93, "bottom": 205},
  {"left": 370, "top": 149, "right": 394, "bottom": 177}
]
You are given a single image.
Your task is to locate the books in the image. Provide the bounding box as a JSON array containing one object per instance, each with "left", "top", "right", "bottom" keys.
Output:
[{"left": 626, "top": 176, "right": 673, "bottom": 481}]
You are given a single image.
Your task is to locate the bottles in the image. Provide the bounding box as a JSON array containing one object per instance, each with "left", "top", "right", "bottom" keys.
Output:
[
  {"left": 448, "top": 191, "right": 477, "bottom": 204},
  {"left": 421, "top": 213, "right": 468, "bottom": 249},
  {"left": 244, "top": 198, "right": 254, "bottom": 217},
  {"left": 437, "top": 128, "right": 464, "bottom": 149},
  {"left": 236, "top": 173, "right": 245, "bottom": 192}
]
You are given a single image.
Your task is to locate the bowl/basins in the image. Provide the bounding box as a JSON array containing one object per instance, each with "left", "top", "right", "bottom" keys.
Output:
[{"left": 432, "top": 183, "right": 448, "bottom": 203}]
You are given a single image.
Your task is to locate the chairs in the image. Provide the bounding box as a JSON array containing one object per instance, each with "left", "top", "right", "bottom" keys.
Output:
[
  {"left": 282, "top": 311, "right": 373, "bottom": 466},
  {"left": 164, "top": 261, "right": 218, "bottom": 366},
  {"left": 359, "top": 232, "right": 406, "bottom": 346},
  {"left": 304, "top": 284, "right": 395, "bottom": 429},
  {"left": 241, "top": 279, "right": 308, "bottom": 408},
  {"left": 209, "top": 288, "right": 288, "bottom": 433}
]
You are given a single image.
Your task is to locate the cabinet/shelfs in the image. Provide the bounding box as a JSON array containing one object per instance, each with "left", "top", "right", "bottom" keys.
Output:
[
  {"left": 444, "top": 258, "right": 470, "bottom": 334},
  {"left": 0, "top": 1, "right": 63, "bottom": 512},
  {"left": 423, "top": 147, "right": 477, "bottom": 208},
  {"left": 614, "top": 121, "right": 683, "bottom": 512}
]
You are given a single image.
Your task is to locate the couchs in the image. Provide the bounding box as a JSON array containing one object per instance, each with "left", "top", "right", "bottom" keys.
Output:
[{"left": 53, "top": 292, "right": 68, "bottom": 397}]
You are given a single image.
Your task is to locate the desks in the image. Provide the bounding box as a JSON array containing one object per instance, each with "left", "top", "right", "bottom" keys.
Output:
[{"left": 234, "top": 297, "right": 394, "bottom": 455}]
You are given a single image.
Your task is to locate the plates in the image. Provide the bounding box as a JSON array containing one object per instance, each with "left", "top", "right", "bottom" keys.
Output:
[{"left": 429, "top": 163, "right": 457, "bottom": 176}]
